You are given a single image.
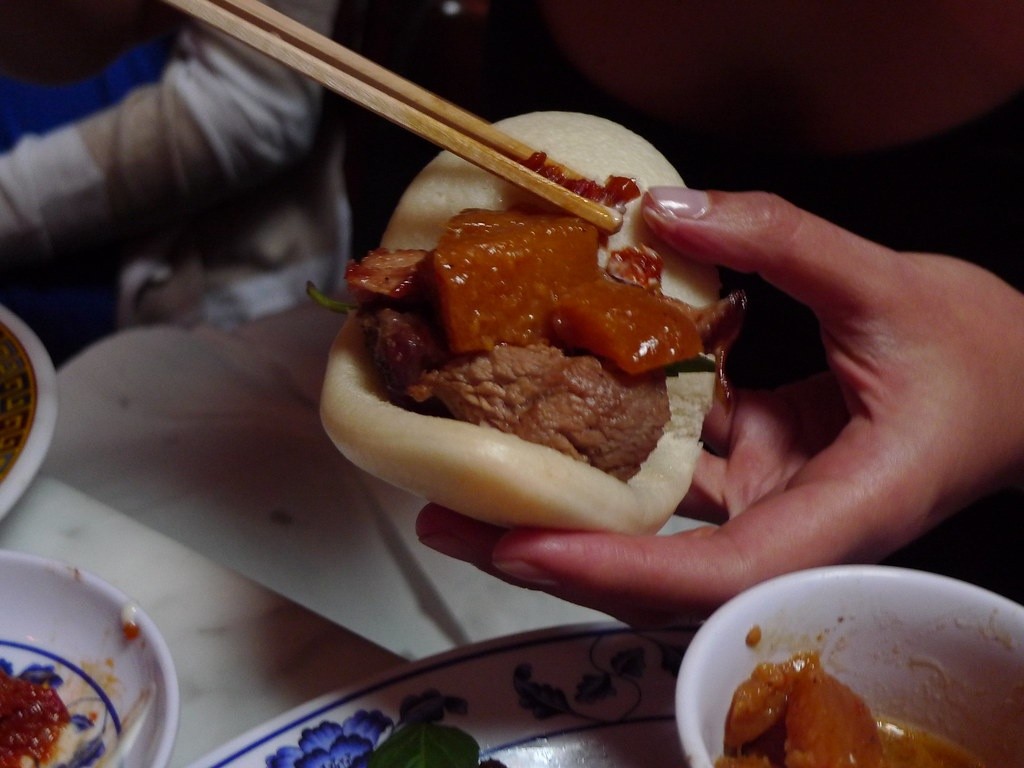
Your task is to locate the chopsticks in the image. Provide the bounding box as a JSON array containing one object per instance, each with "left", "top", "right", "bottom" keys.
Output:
[{"left": 171, "top": 0, "right": 626, "bottom": 235}]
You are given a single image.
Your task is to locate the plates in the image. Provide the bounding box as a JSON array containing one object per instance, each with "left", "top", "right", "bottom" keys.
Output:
[
  {"left": 0, "top": 548, "right": 182, "bottom": 768},
  {"left": 0, "top": 305, "right": 60, "bottom": 524},
  {"left": 186, "top": 621, "right": 708, "bottom": 767}
]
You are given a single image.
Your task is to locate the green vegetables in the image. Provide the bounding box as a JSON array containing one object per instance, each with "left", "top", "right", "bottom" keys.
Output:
[{"left": 367, "top": 718, "right": 481, "bottom": 767}]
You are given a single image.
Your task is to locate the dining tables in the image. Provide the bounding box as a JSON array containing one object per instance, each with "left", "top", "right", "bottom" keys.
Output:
[{"left": 0, "top": 477, "right": 405, "bottom": 768}]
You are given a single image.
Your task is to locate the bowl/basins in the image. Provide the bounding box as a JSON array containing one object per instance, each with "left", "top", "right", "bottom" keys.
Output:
[{"left": 674, "top": 565, "right": 1023, "bottom": 767}]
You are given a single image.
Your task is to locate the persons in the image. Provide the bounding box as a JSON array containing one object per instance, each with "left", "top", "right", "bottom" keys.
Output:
[
  {"left": 372, "top": 0, "right": 1024, "bottom": 634},
  {"left": 0, "top": 0, "right": 356, "bottom": 372}
]
